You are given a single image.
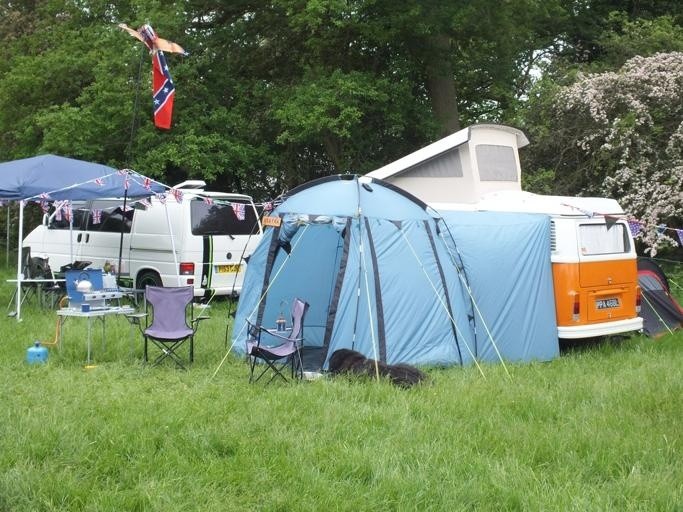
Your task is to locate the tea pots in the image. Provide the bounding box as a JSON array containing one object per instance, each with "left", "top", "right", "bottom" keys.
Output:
[{"left": 72, "top": 271, "right": 94, "bottom": 293}]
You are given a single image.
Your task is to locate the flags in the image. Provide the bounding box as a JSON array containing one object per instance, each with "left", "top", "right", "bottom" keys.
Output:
[{"left": 143, "top": 25, "right": 176, "bottom": 129}]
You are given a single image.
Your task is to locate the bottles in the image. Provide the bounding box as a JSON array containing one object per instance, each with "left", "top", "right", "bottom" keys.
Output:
[
  {"left": 26, "top": 340, "right": 49, "bottom": 365},
  {"left": 276, "top": 311, "right": 286, "bottom": 331}
]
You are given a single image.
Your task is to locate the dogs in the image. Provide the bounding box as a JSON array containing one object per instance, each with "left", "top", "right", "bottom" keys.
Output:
[{"left": 326, "top": 348, "right": 427, "bottom": 391}]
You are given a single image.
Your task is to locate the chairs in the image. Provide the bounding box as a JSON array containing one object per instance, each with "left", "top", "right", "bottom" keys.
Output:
[
  {"left": 240, "top": 297, "right": 311, "bottom": 387},
  {"left": 141, "top": 283, "right": 195, "bottom": 371}
]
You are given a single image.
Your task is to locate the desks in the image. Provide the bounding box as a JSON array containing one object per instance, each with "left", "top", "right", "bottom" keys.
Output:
[
  {"left": 5, "top": 277, "right": 65, "bottom": 321},
  {"left": 54, "top": 307, "right": 135, "bottom": 366}
]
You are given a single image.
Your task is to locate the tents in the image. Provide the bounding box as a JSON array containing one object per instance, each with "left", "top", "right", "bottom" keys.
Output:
[
  {"left": 634, "top": 257, "right": 683, "bottom": 340},
  {"left": 229, "top": 175, "right": 559, "bottom": 377}
]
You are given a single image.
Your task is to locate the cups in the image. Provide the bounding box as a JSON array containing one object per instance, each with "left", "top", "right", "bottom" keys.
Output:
[{"left": 81, "top": 303, "right": 90, "bottom": 313}]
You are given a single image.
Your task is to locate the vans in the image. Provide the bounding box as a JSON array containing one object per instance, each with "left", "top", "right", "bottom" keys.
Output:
[{"left": 22, "top": 180, "right": 264, "bottom": 300}]
